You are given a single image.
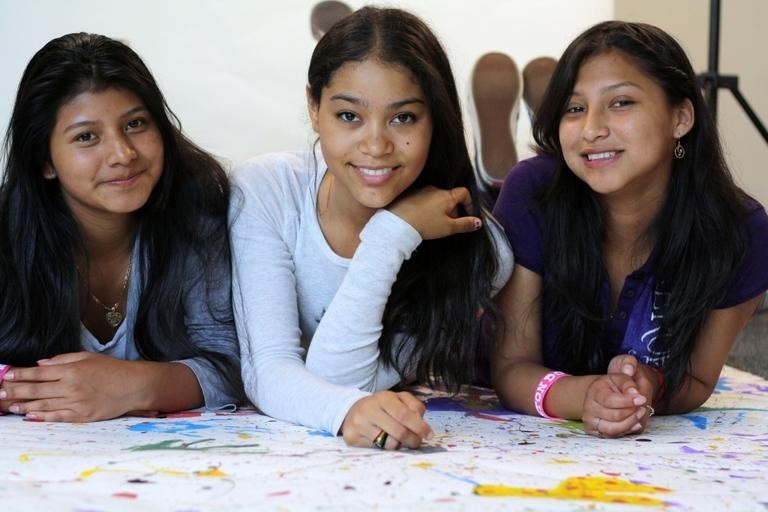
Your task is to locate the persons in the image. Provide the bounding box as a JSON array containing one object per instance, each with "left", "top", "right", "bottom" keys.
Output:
[
  {"left": 225, "top": 6, "right": 516, "bottom": 451},
  {"left": 0, "top": 32, "right": 244, "bottom": 424},
  {"left": 466, "top": 20, "right": 768, "bottom": 440}
]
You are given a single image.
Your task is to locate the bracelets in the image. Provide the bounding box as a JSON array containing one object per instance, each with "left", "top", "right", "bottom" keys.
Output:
[
  {"left": 533, "top": 369, "right": 573, "bottom": 422},
  {"left": 644, "top": 366, "right": 664, "bottom": 402}
]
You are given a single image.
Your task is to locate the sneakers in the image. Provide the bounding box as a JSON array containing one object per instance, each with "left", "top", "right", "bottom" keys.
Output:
[
  {"left": 467, "top": 52, "right": 525, "bottom": 193},
  {"left": 310, "top": 0, "right": 352, "bottom": 40},
  {"left": 522, "top": 57, "right": 560, "bottom": 123}
]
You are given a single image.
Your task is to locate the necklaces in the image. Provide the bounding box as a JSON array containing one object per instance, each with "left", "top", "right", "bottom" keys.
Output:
[{"left": 67, "top": 244, "right": 132, "bottom": 328}]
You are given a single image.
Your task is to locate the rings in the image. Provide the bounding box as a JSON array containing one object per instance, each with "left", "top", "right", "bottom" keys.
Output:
[
  {"left": 589, "top": 415, "right": 604, "bottom": 438},
  {"left": 642, "top": 404, "right": 655, "bottom": 419},
  {"left": 373, "top": 429, "right": 389, "bottom": 448}
]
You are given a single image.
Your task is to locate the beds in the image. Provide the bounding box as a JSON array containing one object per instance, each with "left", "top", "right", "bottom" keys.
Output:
[{"left": 0, "top": 363, "right": 768, "bottom": 512}]
